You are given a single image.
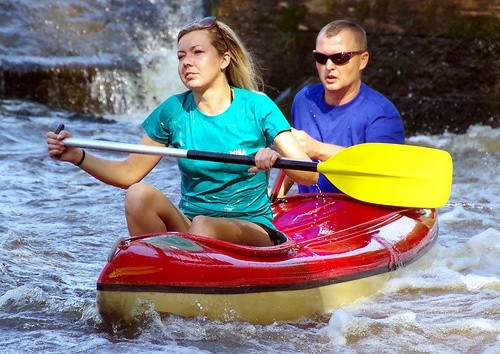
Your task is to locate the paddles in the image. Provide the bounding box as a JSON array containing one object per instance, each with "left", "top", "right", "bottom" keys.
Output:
[{"left": 52, "top": 124, "right": 452, "bottom": 208}]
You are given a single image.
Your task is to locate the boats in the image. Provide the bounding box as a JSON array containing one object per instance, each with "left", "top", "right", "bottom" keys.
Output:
[{"left": 95, "top": 191, "right": 439, "bottom": 326}]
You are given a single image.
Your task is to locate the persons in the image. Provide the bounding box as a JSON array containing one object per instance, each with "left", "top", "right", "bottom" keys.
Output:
[
  {"left": 47, "top": 17, "right": 319, "bottom": 247},
  {"left": 266, "top": 20, "right": 404, "bottom": 199}
]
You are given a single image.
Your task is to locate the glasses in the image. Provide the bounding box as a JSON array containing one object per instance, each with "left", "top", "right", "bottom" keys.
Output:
[
  {"left": 181, "top": 16, "right": 228, "bottom": 52},
  {"left": 312, "top": 50, "right": 366, "bottom": 65}
]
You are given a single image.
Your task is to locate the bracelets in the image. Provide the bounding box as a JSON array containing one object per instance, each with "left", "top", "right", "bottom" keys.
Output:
[{"left": 73, "top": 149, "right": 86, "bottom": 166}]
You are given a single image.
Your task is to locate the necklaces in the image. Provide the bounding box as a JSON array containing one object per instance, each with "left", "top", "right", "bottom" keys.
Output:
[{"left": 229, "top": 88, "right": 233, "bottom": 104}]
